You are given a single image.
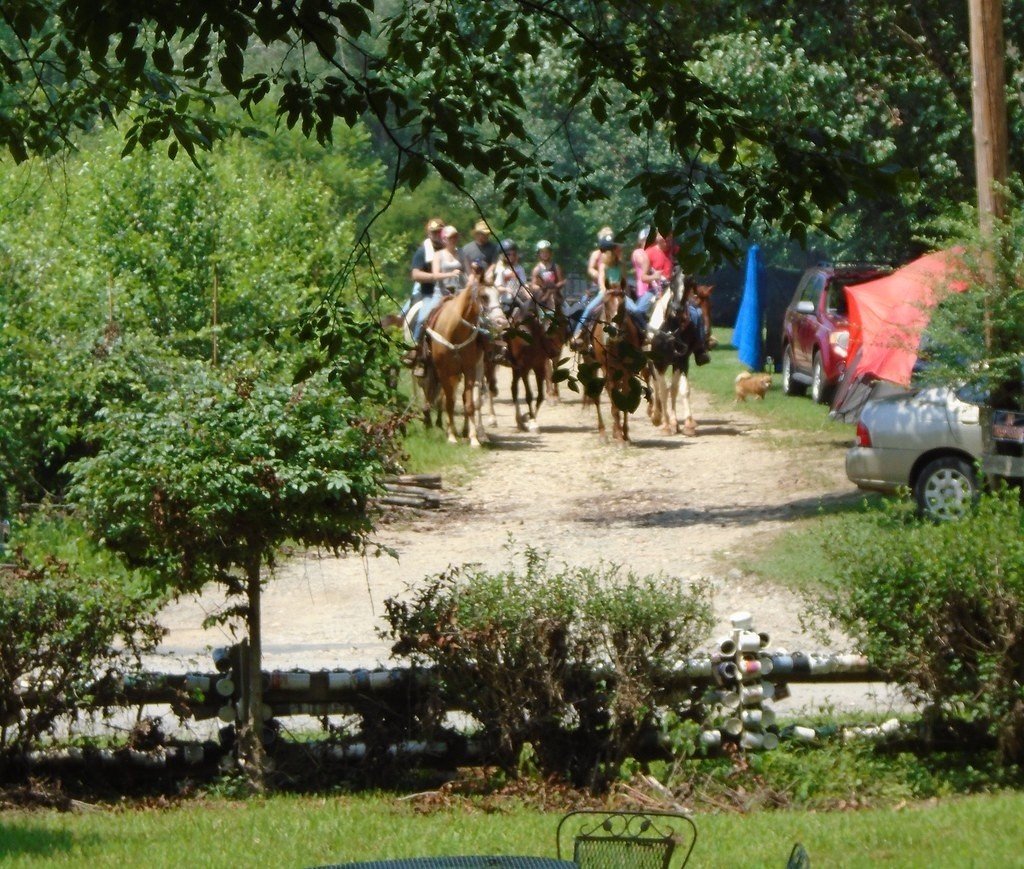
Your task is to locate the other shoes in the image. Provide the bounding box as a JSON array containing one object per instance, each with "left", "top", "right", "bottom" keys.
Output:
[
  {"left": 570, "top": 337, "right": 584, "bottom": 350},
  {"left": 413, "top": 358, "right": 425, "bottom": 376},
  {"left": 705, "top": 339, "right": 716, "bottom": 350},
  {"left": 694, "top": 349, "right": 710, "bottom": 365},
  {"left": 401, "top": 352, "right": 416, "bottom": 369}
]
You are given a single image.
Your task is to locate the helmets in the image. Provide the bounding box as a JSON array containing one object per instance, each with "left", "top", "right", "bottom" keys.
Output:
[
  {"left": 496, "top": 239, "right": 519, "bottom": 254},
  {"left": 536, "top": 240, "right": 551, "bottom": 253}
]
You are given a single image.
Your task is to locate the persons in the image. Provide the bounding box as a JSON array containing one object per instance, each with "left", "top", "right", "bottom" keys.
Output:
[
  {"left": 484, "top": 239, "right": 529, "bottom": 317},
  {"left": 529, "top": 240, "right": 577, "bottom": 334},
  {"left": 396, "top": 219, "right": 483, "bottom": 376},
  {"left": 570, "top": 226, "right": 649, "bottom": 353},
  {"left": 632, "top": 225, "right": 718, "bottom": 367},
  {"left": 460, "top": 221, "right": 499, "bottom": 288}
]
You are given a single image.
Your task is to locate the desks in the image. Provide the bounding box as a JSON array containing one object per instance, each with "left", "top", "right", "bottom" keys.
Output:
[{"left": 307, "top": 855, "right": 581, "bottom": 869}]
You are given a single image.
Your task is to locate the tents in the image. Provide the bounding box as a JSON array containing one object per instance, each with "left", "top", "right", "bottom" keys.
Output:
[
  {"left": 731, "top": 230, "right": 831, "bottom": 376},
  {"left": 832, "top": 239, "right": 999, "bottom": 424}
]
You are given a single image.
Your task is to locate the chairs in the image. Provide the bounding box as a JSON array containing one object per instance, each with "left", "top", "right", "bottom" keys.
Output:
[{"left": 555, "top": 809, "right": 697, "bottom": 869}]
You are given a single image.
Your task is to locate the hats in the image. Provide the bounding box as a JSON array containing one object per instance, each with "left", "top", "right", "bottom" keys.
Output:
[
  {"left": 599, "top": 235, "right": 627, "bottom": 249},
  {"left": 639, "top": 228, "right": 650, "bottom": 240},
  {"left": 427, "top": 219, "right": 444, "bottom": 231},
  {"left": 440, "top": 225, "right": 458, "bottom": 238},
  {"left": 471, "top": 221, "right": 491, "bottom": 236}
]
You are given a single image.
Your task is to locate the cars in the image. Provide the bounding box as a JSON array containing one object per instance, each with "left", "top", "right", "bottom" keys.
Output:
[
  {"left": 843, "top": 350, "right": 1024, "bottom": 523},
  {"left": 781, "top": 267, "right": 900, "bottom": 403}
]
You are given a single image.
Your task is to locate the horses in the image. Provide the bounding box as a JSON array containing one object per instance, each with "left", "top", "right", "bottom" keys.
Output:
[{"left": 378, "top": 265, "right": 702, "bottom": 450}]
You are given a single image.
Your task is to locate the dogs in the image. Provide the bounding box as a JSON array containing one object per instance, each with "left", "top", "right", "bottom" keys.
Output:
[{"left": 732, "top": 370, "right": 773, "bottom": 405}]
